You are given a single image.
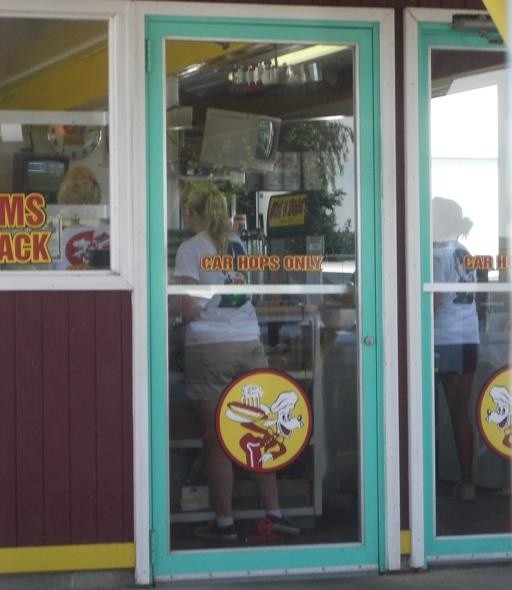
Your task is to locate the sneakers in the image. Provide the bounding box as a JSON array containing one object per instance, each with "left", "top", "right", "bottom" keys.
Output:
[
  {"left": 266, "top": 512, "right": 301, "bottom": 536},
  {"left": 193, "top": 520, "right": 236, "bottom": 543},
  {"left": 450, "top": 482, "right": 476, "bottom": 500}
]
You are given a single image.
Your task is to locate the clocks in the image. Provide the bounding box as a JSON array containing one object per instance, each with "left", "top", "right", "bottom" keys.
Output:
[{"left": 46, "top": 124, "right": 101, "bottom": 160}]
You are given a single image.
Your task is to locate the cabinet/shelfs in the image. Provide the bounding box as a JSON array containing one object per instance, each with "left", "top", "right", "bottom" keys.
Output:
[{"left": 170, "top": 304, "right": 323, "bottom": 523}]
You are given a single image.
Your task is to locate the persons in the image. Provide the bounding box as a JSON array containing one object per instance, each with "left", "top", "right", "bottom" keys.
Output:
[
  {"left": 54, "top": 167, "right": 107, "bottom": 234},
  {"left": 432, "top": 189, "right": 482, "bottom": 501},
  {"left": 167, "top": 172, "right": 289, "bottom": 542}
]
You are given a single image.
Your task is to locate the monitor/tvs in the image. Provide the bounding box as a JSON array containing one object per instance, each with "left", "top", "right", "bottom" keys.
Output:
[{"left": 13, "top": 152, "right": 69, "bottom": 204}]
